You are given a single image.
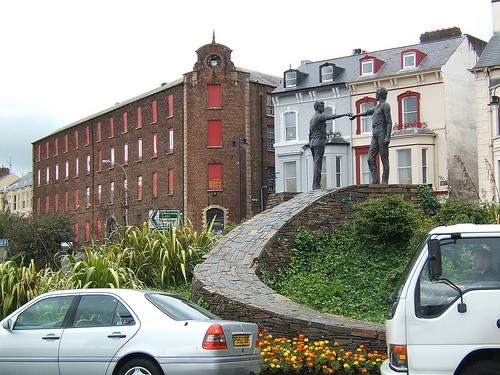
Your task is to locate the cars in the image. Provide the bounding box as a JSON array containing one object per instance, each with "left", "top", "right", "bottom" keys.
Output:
[{"left": 0, "top": 288, "right": 263, "bottom": 375}]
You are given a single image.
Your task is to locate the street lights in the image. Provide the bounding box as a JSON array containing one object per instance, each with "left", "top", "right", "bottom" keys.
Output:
[{"left": 102, "top": 159, "right": 129, "bottom": 225}]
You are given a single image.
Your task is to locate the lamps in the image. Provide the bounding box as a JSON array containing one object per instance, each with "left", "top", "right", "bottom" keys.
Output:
[
  {"left": 354, "top": 49, "right": 362, "bottom": 55},
  {"left": 242, "top": 138, "right": 248, "bottom": 146},
  {"left": 488, "top": 85, "right": 500, "bottom": 108},
  {"left": 231, "top": 140, "right": 238, "bottom": 147}
]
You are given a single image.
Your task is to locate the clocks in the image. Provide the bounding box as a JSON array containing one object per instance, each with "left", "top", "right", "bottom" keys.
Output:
[{"left": 205, "top": 53, "right": 223, "bottom": 70}]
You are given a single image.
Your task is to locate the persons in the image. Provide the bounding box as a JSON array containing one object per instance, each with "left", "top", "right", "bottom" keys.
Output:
[
  {"left": 62, "top": 247, "right": 74, "bottom": 273},
  {"left": 349, "top": 87, "right": 392, "bottom": 184},
  {"left": 309, "top": 100, "right": 353, "bottom": 190},
  {"left": 463, "top": 248, "right": 500, "bottom": 287}
]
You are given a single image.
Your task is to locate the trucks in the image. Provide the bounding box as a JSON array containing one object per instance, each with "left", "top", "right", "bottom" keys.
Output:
[{"left": 379, "top": 224, "right": 500, "bottom": 375}]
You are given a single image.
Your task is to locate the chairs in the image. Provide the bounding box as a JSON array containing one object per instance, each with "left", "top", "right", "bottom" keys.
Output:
[{"left": 76, "top": 300, "right": 110, "bottom": 327}]
